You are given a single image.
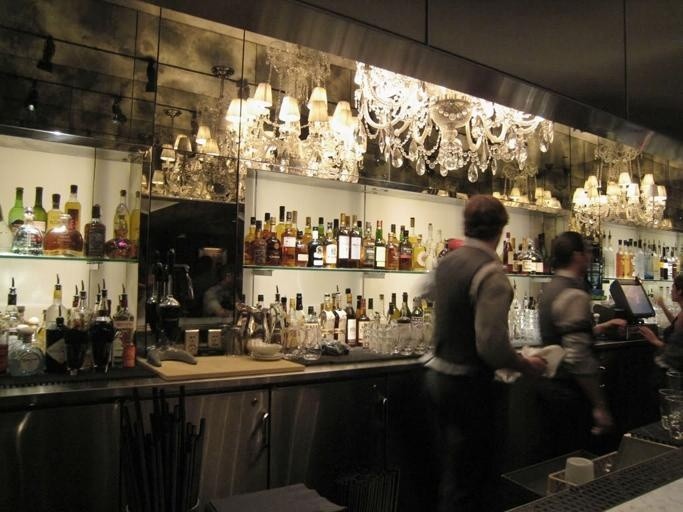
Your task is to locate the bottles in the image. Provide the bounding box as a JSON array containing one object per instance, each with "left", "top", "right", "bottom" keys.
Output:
[
  {"left": 158, "top": 249, "right": 180, "bottom": 332},
  {"left": 498, "top": 232, "right": 553, "bottom": 276},
  {"left": 523, "top": 295, "right": 538, "bottom": 310},
  {"left": 0, "top": 180, "right": 105, "bottom": 259},
  {"left": 600, "top": 230, "right": 681, "bottom": 279},
  {"left": 113, "top": 188, "right": 139, "bottom": 245},
  {"left": 146, "top": 251, "right": 160, "bottom": 332},
  {"left": 281, "top": 329, "right": 301, "bottom": 360},
  {"left": 301, "top": 325, "right": 324, "bottom": 359},
  {"left": 241, "top": 287, "right": 434, "bottom": 343},
  {"left": 0, "top": 273, "right": 137, "bottom": 377},
  {"left": 240, "top": 203, "right": 445, "bottom": 271},
  {"left": 645, "top": 285, "right": 672, "bottom": 302}
]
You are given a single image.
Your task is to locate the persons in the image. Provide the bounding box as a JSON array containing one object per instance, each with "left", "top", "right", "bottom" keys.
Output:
[
  {"left": 201, "top": 264, "right": 241, "bottom": 317},
  {"left": 536, "top": 230, "right": 629, "bottom": 447},
  {"left": 631, "top": 271, "right": 683, "bottom": 400},
  {"left": 417, "top": 193, "right": 548, "bottom": 511}
]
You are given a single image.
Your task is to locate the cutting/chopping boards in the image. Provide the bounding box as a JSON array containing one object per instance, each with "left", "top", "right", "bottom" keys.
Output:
[{"left": 136, "top": 354, "right": 305, "bottom": 381}]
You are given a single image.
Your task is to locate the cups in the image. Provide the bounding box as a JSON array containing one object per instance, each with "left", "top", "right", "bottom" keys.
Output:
[
  {"left": 506, "top": 310, "right": 542, "bottom": 346},
  {"left": 564, "top": 456, "right": 594, "bottom": 484},
  {"left": 658, "top": 369, "right": 682, "bottom": 441},
  {"left": 652, "top": 303, "right": 680, "bottom": 327},
  {"left": 357, "top": 320, "right": 430, "bottom": 356}
]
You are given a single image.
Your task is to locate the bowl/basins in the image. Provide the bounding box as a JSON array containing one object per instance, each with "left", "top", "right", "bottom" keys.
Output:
[{"left": 250, "top": 344, "right": 282, "bottom": 357}]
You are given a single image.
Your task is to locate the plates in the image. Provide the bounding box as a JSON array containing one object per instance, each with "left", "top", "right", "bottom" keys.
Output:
[{"left": 248, "top": 352, "right": 282, "bottom": 360}]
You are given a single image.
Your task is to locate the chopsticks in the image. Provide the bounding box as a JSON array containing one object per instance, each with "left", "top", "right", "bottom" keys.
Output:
[{"left": 115, "top": 383, "right": 206, "bottom": 511}]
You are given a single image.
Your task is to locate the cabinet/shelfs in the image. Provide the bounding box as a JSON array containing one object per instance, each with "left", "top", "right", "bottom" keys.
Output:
[
  {"left": 270, "top": 379, "right": 372, "bottom": 486},
  {"left": 1, "top": 0, "right": 682, "bottom": 373},
  {"left": 124, "top": 388, "right": 269, "bottom": 498},
  {"left": 1, "top": 402, "right": 123, "bottom": 512}
]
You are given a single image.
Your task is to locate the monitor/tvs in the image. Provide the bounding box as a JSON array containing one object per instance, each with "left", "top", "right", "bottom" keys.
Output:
[{"left": 609, "top": 278, "right": 655, "bottom": 318}]
